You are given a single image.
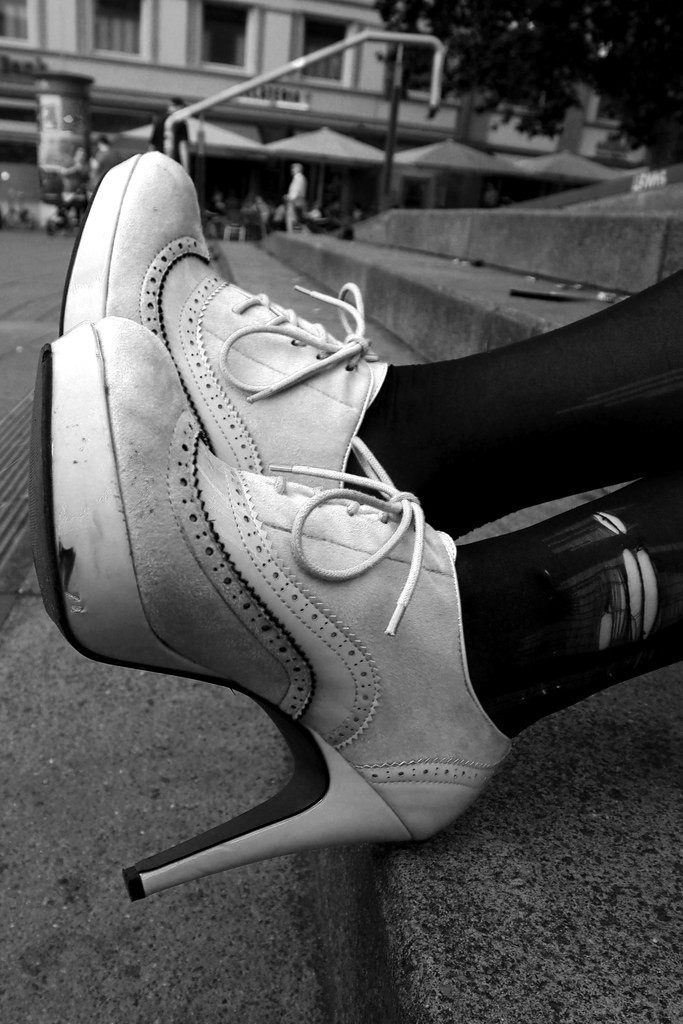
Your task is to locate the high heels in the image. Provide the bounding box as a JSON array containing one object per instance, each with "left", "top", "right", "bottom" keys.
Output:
[
  {"left": 61, "top": 150, "right": 392, "bottom": 490},
  {"left": 30, "top": 315, "right": 513, "bottom": 902}
]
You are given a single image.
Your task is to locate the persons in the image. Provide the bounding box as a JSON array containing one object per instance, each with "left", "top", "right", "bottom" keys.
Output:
[
  {"left": 64, "top": 135, "right": 126, "bottom": 226},
  {"left": 29, "top": 153, "right": 683, "bottom": 902},
  {"left": 148, "top": 98, "right": 188, "bottom": 171},
  {"left": 204, "top": 162, "right": 498, "bottom": 241}
]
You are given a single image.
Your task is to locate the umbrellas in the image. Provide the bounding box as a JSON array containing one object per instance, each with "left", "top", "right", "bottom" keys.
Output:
[
  {"left": 121, "top": 117, "right": 266, "bottom": 157},
  {"left": 267, "top": 126, "right": 389, "bottom": 209},
  {"left": 394, "top": 138, "right": 627, "bottom": 207}
]
